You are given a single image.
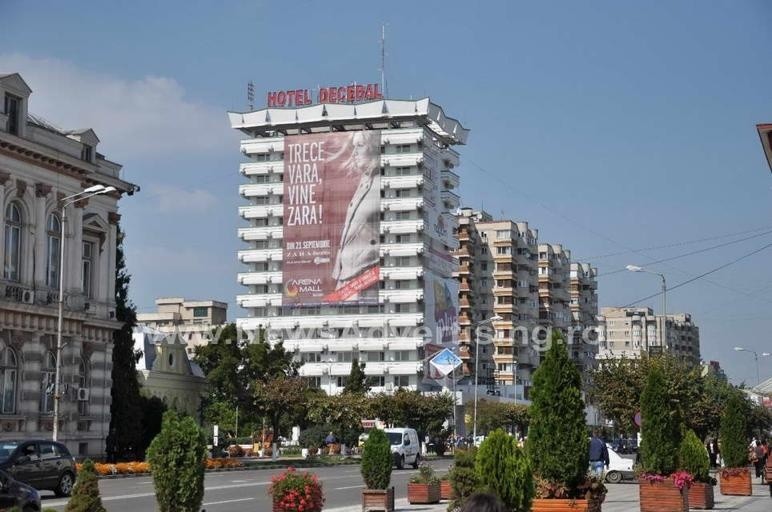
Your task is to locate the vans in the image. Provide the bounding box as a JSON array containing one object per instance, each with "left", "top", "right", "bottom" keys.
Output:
[{"left": 383, "top": 427, "right": 421, "bottom": 469}]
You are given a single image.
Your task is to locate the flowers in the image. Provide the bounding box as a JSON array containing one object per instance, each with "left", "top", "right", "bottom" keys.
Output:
[{"left": 270, "top": 466, "right": 325, "bottom": 512}]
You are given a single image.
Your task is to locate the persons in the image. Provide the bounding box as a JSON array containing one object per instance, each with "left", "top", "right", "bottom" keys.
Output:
[
  {"left": 325, "top": 432, "right": 336, "bottom": 456},
  {"left": 589, "top": 429, "right": 609, "bottom": 484},
  {"left": 707, "top": 438, "right": 720, "bottom": 467},
  {"left": 327, "top": 131, "right": 380, "bottom": 302},
  {"left": 748, "top": 437, "right": 769, "bottom": 478}
]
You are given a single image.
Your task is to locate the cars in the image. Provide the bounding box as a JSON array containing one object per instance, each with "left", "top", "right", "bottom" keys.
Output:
[
  {"left": 0, "top": 470, "right": 41, "bottom": 512},
  {"left": 586, "top": 437, "right": 638, "bottom": 483},
  {"left": 422, "top": 435, "right": 527, "bottom": 456}
]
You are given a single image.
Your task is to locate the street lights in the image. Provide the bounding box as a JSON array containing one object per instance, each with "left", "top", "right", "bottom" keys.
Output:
[
  {"left": 473, "top": 316, "right": 501, "bottom": 446},
  {"left": 733, "top": 347, "right": 772, "bottom": 409},
  {"left": 51, "top": 185, "right": 117, "bottom": 458},
  {"left": 625, "top": 265, "right": 667, "bottom": 355}
]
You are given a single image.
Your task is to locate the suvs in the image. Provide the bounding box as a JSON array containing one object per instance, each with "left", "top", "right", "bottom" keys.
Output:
[{"left": 0, "top": 438, "right": 77, "bottom": 497}]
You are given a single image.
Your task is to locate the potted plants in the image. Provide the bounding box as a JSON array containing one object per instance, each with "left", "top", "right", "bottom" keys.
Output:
[
  {"left": 530, "top": 351, "right": 754, "bottom": 510},
  {"left": 359, "top": 429, "right": 454, "bottom": 512}
]
